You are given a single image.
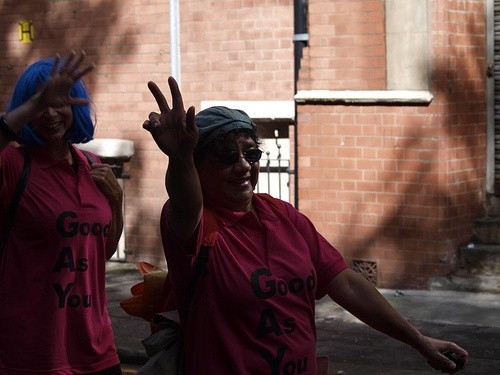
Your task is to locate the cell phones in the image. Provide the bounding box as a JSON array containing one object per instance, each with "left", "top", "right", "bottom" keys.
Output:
[{"left": 426, "top": 351, "right": 464, "bottom": 370}]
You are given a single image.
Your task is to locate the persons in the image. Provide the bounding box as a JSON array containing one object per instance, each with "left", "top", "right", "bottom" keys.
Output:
[
  {"left": 142, "top": 77, "right": 468, "bottom": 375},
  {"left": 0, "top": 49, "right": 123, "bottom": 375}
]
